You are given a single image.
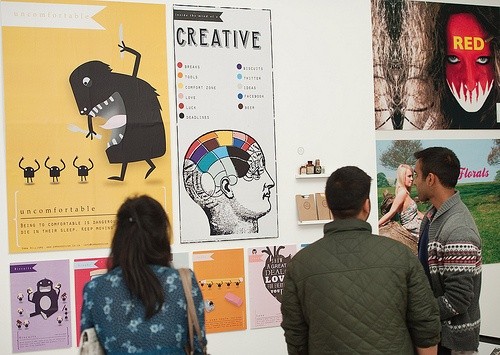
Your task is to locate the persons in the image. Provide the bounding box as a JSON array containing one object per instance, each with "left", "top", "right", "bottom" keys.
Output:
[
  {"left": 280, "top": 166, "right": 442, "bottom": 355},
  {"left": 80, "top": 193, "right": 209, "bottom": 355},
  {"left": 378, "top": 164, "right": 424, "bottom": 243},
  {"left": 412, "top": 146, "right": 482, "bottom": 355}
]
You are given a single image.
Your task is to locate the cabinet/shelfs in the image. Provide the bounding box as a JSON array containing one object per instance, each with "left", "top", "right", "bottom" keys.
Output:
[{"left": 296, "top": 174, "right": 334, "bottom": 225}]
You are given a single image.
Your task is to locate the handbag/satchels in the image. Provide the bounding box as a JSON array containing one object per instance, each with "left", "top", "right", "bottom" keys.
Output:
[{"left": 76, "top": 328, "right": 105, "bottom": 355}]
